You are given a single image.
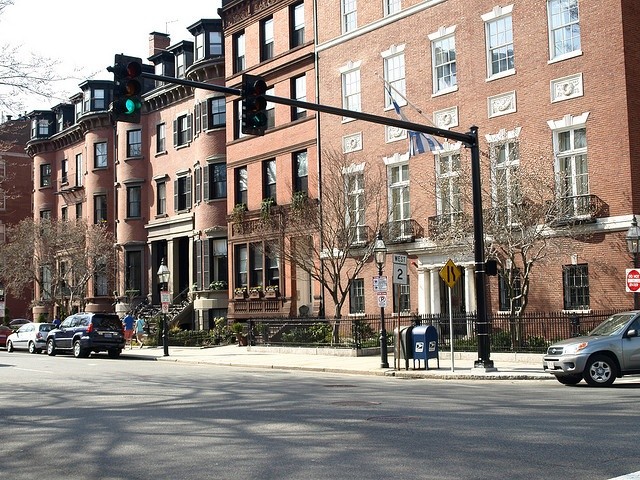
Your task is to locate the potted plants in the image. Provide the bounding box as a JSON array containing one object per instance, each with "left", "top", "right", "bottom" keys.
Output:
[
  {"left": 210, "top": 280, "right": 226, "bottom": 290},
  {"left": 233, "top": 322, "right": 243, "bottom": 342}
]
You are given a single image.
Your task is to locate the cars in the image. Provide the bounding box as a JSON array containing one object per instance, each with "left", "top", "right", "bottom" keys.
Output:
[
  {"left": 1, "top": 319, "right": 32, "bottom": 331},
  {"left": 6, "top": 323, "right": 65, "bottom": 354},
  {"left": 0, "top": 326, "right": 15, "bottom": 347}
]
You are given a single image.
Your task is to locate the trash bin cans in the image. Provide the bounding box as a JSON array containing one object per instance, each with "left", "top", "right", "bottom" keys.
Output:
[{"left": 394, "top": 325, "right": 439, "bottom": 369}]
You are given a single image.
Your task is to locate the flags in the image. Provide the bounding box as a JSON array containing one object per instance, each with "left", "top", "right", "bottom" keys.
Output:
[{"left": 383, "top": 83, "right": 443, "bottom": 159}]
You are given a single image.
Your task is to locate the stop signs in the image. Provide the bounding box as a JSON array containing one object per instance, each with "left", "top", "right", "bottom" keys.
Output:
[{"left": 626, "top": 268, "right": 640, "bottom": 293}]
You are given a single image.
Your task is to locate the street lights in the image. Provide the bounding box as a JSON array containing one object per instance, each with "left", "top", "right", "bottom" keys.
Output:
[
  {"left": 625, "top": 215, "right": 640, "bottom": 309},
  {"left": 372, "top": 230, "right": 389, "bottom": 368},
  {"left": 157, "top": 258, "right": 170, "bottom": 357}
]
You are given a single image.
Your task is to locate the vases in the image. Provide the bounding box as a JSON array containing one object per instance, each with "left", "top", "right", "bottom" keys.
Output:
[
  {"left": 234, "top": 293, "right": 245, "bottom": 299},
  {"left": 266, "top": 292, "right": 277, "bottom": 298},
  {"left": 250, "top": 292, "right": 261, "bottom": 299}
]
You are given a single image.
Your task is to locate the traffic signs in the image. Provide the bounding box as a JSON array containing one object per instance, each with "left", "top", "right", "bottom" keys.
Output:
[{"left": 393, "top": 252, "right": 407, "bottom": 284}]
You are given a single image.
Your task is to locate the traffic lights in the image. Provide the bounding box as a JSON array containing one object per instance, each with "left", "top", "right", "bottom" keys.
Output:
[
  {"left": 241, "top": 73, "right": 267, "bottom": 137},
  {"left": 112, "top": 54, "right": 142, "bottom": 125}
]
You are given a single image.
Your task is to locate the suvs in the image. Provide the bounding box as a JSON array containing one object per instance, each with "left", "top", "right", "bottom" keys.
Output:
[
  {"left": 46, "top": 312, "right": 126, "bottom": 358},
  {"left": 543, "top": 310, "right": 640, "bottom": 387}
]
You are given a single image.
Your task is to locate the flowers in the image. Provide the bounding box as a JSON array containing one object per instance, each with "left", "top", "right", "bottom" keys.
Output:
[
  {"left": 266, "top": 285, "right": 278, "bottom": 291},
  {"left": 234, "top": 287, "right": 246, "bottom": 294},
  {"left": 249, "top": 286, "right": 261, "bottom": 292}
]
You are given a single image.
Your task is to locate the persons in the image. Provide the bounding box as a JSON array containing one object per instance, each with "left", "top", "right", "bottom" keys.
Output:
[
  {"left": 122, "top": 311, "right": 133, "bottom": 349},
  {"left": 52, "top": 315, "right": 61, "bottom": 326},
  {"left": 135, "top": 314, "right": 146, "bottom": 349}
]
